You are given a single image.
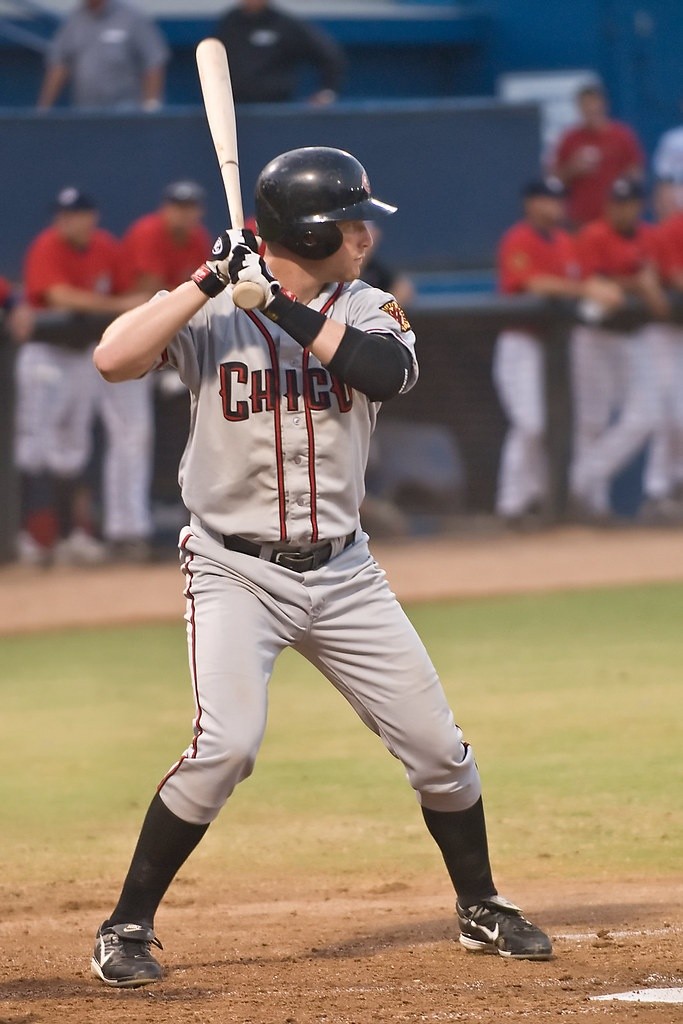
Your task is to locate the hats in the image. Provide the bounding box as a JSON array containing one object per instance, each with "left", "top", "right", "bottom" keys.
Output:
[
  {"left": 50, "top": 189, "right": 97, "bottom": 212},
  {"left": 523, "top": 177, "right": 567, "bottom": 198},
  {"left": 163, "top": 182, "right": 207, "bottom": 203},
  {"left": 610, "top": 180, "right": 643, "bottom": 199}
]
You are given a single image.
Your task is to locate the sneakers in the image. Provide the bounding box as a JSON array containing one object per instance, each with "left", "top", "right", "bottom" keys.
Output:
[
  {"left": 456, "top": 895, "right": 553, "bottom": 960},
  {"left": 89, "top": 920, "right": 163, "bottom": 984}
]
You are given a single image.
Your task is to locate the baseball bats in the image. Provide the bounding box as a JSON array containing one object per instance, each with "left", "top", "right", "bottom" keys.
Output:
[{"left": 188, "top": 34, "right": 268, "bottom": 308}]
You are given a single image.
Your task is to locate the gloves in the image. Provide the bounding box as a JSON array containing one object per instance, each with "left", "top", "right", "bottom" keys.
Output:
[
  {"left": 228, "top": 246, "right": 298, "bottom": 321},
  {"left": 191, "top": 226, "right": 262, "bottom": 296}
]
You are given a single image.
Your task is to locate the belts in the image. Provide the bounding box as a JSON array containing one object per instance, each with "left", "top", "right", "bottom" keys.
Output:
[{"left": 224, "top": 530, "right": 356, "bottom": 571}]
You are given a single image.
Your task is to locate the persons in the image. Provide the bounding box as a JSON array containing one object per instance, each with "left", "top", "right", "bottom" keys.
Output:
[
  {"left": 0, "top": 1, "right": 683, "bottom": 572},
  {"left": 86, "top": 145, "right": 554, "bottom": 986}
]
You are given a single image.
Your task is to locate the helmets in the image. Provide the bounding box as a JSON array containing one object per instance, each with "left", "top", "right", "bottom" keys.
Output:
[{"left": 255, "top": 145, "right": 397, "bottom": 259}]
